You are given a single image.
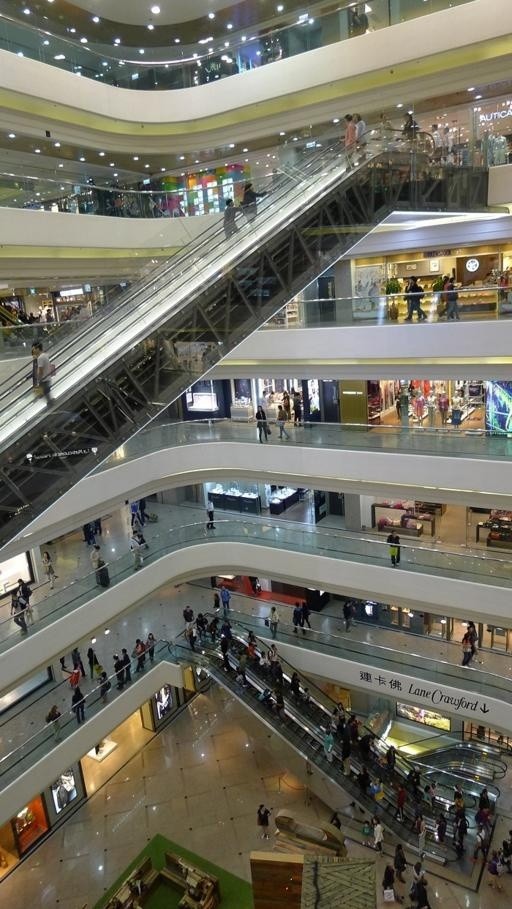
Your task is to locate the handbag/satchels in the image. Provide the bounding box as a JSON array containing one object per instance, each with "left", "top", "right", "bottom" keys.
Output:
[
  {"left": 23, "top": 587, "right": 32, "bottom": 597},
  {"left": 266, "top": 426, "right": 271, "bottom": 435},
  {"left": 375, "top": 792, "right": 385, "bottom": 802},
  {"left": 49, "top": 364, "right": 56, "bottom": 376},
  {"left": 383, "top": 889, "right": 395, "bottom": 902},
  {"left": 389, "top": 546, "right": 398, "bottom": 556}
]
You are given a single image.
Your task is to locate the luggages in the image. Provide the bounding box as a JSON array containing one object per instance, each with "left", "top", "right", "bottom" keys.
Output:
[
  {"left": 98, "top": 567, "right": 110, "bottom": 587},
  {"left": 146, "top": 512, "right": 159, "bottom": 520}
]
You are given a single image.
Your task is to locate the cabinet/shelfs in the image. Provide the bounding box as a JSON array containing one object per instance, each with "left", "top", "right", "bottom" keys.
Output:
[
  {"left": 390, "top": 285, "right": 498, "bottom": 315},
  {"left": 210, "top": 482, "right": 301, "bottom": 515},
  {"left": 231, "top": 399, "right": 253, "bottom": 422},
  {"left": 371, "top": 499, "right": 512, "bottom": 548},
  {"left": 97, "top": 854, "right": 221, "bottom": 909}
]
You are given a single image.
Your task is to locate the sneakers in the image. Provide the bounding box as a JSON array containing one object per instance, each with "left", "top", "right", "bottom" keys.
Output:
[{"left": 391, "top": 562, "right": 400, "bottom": 566}]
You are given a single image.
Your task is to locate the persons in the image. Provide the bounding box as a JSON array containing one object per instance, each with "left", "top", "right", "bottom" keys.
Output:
[
  {"left": 9, "top": 591, "right": 30, "bottom": 636},
  {"left": 442, "top": 126, "right": 453, "bottom": 153},
  {"left": 180, "top": 585, "right": 312, "bottom": 722},
  {"left": 32, "top": 342, "right": 52, "bottom": 406},
  {"left": 438, "top": 276, "right": 451, "bottom": 321},
  {"left": 256, "top": 386, "right": 303, "bottom": 444},
  {"left": 402, "top": 113, "right": 419, "bottom": 143},
  {"left": 461, "top": 619, "right": 481, "bottom": 669},
  {"left": 387, "top": 530, "right": 400, "bottom": 568},
  {"left": 341, "top": 599, "right": 355, "bottom": 633},
  {"left": 379, "top": 113, "right": 395, "bottom": 142},
  {"left": 431, "top": 124, "right": 441, "bottom": 151},
  {"left": 257, "top": 804, "right": 272, "bottom": 839},
  {"left": 239, "top": 183, "right": 271, "bottom": 222},
  {"left": 206, "top": 499, "right": 216, "bottom": 529},
  {"left": 223, "top": 199, "right": 254, "bottom": 238},
  {"left": 322, "top": 701, "right": 511, "bottom": 907},
  {"left": 446, "top": 278, "right": 460, "bottom": 322},
  {"left": 90, "top": 545, "right": 102, "bottom": 584},
  {"left": 340, "top": 114, "right": 355, "bottom": 172},
  {"left": 41, "top": 552, "right": 56, "bottom": 590},
  {"left": 16, "top": 579, "right": 32, "bottom": 609},
  {"left": 17, "top": 307, "right": 83, "bottom": 323},
  {"left": 404, "top": 276, "right": 427, "bottom": 322},
  {"left": 129, "top": 497, "right": 150, "bottom": 550},
  {"left": 413, "top": 390, "right": 463, "bottom": 429},
  {"left": 45, "top": 633, "right": 156, "bottom": 741},
  {"left": 352, "top": 113, "right": 366, "bottom": 165}
]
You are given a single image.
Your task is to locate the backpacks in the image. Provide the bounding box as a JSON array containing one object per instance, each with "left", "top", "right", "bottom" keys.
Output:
[{"left": 496, "top": 860, "right": 506, "bottom": 876}]
